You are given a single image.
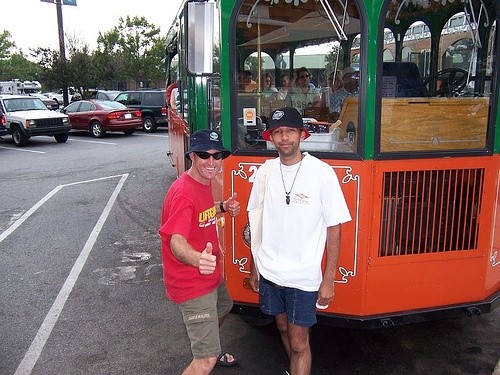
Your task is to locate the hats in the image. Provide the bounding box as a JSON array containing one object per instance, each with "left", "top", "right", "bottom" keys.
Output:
[
  {"left": 262, "top": 107, "right": 311, "bottom": 142},
  {"left": 184, "top": 129, "right": 231, "bottom": 161},
  {"left": 339, "top": 66, "right": 359, "bottom": 80}
]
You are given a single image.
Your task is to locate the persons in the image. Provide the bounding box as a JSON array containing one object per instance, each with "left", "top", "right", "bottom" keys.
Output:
[
  {"left": 289, "top": 66, "right": 321, "bottom": 116},
  {"left": 246, "top": 107, "right": 352, "bottom": 375},
  {"left": 159, "top": 129, "right": 241, "bottom": 375},
  {"left": 322, "top": 70, "right": 342, "bottom": 107},
  {"left": 329, "top": 66, "right": 360, "bottom": 122},
  {"left": 279, "top": 74, "right": 290, "bottom": 100},
  {"left": 243, "top": 70, "right": 258, "bottom": 92},
  {"left": 261, "top": 73, "right": 278, "bottom": 91}
]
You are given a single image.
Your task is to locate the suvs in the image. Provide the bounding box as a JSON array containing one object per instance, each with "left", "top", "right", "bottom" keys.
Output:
[{"left": 113, "top": 88, "right": 170, "bottom": 133}]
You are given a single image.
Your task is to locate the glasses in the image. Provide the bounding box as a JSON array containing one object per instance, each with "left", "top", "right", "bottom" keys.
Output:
[
  {"left": 299, "top": 75, "right": 310, "bottom": 78},
  {"left": 193, "top": 150, "right": 224, "bottom": 160}
]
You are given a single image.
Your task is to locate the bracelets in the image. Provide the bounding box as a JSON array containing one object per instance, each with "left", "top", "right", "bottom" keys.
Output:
[{"left": 220, "top": 202, "right": 225, "bottom": 213}]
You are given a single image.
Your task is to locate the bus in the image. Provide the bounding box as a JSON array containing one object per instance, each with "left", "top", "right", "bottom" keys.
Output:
[{"left": 163, "top": 0, "right": 497, "bottom": 328}]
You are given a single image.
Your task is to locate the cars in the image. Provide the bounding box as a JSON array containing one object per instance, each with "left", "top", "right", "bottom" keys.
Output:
[
  {"left": 0, "top": 95, "right": 72, "bottom": 148},
  {"left": 48, "top": 86, "right": 127, "bottom": 105},
  {"left": 60, "top": 99, "right": 143, "bottom": 138},
  {"left": 25, "top": 93, "right": 58, "bottom": 110}
]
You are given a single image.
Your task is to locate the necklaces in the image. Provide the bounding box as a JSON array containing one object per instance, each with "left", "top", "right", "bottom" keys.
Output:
[{"left": 280, "top": 160, "right": 302, "bottom": 205}]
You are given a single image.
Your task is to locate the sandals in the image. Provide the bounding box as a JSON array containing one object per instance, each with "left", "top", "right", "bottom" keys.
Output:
[{"left": 217, "top": 352, "right": 238, "bottom": 366}]
